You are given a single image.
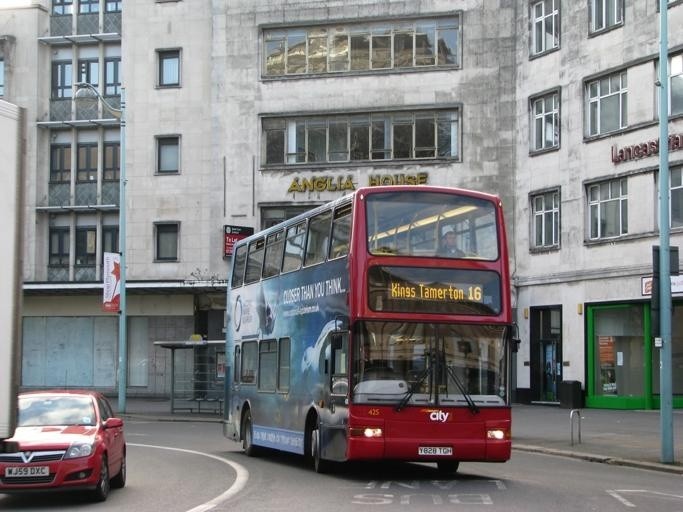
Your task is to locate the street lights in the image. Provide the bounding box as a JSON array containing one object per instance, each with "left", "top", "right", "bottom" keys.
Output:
[{"left": 72, "top": 79, "right": 130, "bottom": 417}]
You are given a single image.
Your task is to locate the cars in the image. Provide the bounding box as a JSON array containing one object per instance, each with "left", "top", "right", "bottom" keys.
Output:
[{"left": 0, "top": 387, "right": 127, "bottom": 503}]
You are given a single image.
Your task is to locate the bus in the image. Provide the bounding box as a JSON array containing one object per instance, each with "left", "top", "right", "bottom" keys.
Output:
[{"left": 223, "top": 183, "right": 514, "bottom": 479}]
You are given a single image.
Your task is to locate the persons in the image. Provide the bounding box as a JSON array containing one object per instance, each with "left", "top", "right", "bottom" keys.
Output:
[{"left": 434, "top": 230, "right": 465, "bottom": 258}]
